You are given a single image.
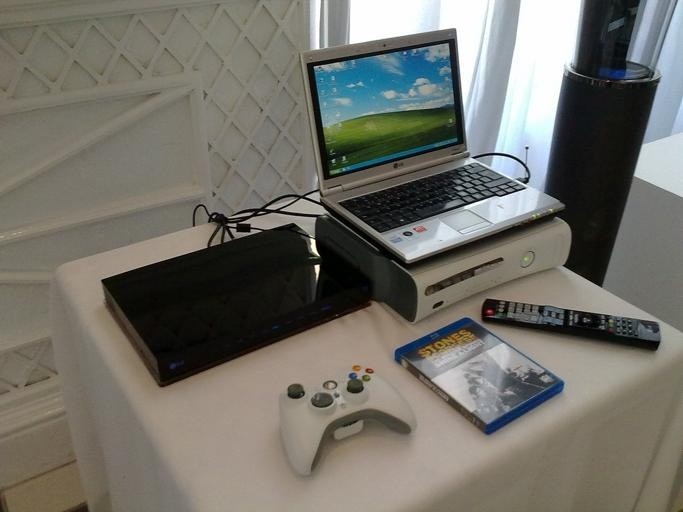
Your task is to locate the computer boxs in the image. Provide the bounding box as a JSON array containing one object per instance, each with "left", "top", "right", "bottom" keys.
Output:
[{"left": 314, "top": 213, "right": 573, "bottom": 321}]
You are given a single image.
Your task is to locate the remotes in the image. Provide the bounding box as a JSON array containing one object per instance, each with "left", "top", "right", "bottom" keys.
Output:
[{"left": 482, "top": 298, "right": 662, "bottom": 351}]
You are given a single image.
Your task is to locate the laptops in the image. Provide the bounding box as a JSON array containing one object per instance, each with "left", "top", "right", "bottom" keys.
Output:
[
  {"left": 101, "top": 223, "right": 373, "bottom": 387},
  {"left": 299, "top": 28, "right": 565, "bottom": 266}
]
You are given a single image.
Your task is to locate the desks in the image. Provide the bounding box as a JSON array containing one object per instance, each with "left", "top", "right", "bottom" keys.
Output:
[{"left": 51, "top": 192, "right": 683, "bottom": 512}]
